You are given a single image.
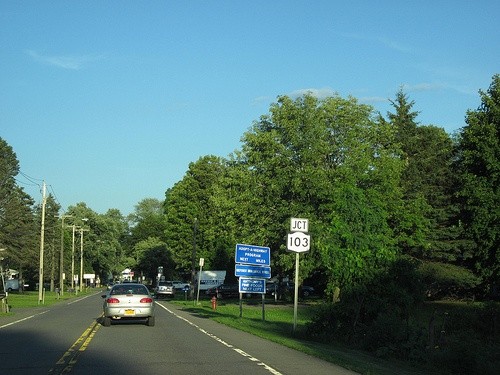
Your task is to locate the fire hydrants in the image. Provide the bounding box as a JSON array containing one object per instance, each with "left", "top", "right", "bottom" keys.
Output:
[{"left": 210, "top": 296, "right": 217, "bottom": 309}]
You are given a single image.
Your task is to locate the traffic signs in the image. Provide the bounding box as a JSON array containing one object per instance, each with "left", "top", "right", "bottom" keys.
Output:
[{"left": 287, "top": 217, "right": 310, "bottom": 252}]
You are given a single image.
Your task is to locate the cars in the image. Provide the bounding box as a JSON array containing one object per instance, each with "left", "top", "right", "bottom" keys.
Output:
[
  {"left": 151, "top": 281, "right": 191, "bottom": 291},
  {"left": 105, "top": 280, "right": 140, "bottom": 286},
  {"left": 155, "top": 279, "right": 175, "bottom": 299},
  {"left": 101, "top": 282, "right": 157, "bottom": 328},
  {"left": 265, "top": 280, "right": 315, "bottom": 297},
  {"left": 207, "top": 282, "right": 258, "bottom": 300}
]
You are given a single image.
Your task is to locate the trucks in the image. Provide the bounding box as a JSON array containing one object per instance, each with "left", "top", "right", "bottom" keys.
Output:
[
  {"left": 3, "top": 279, "right": 23, "bottom": 292},
  {"left": 193, "top": 270, "right": 226, "bottom": 291}
]
